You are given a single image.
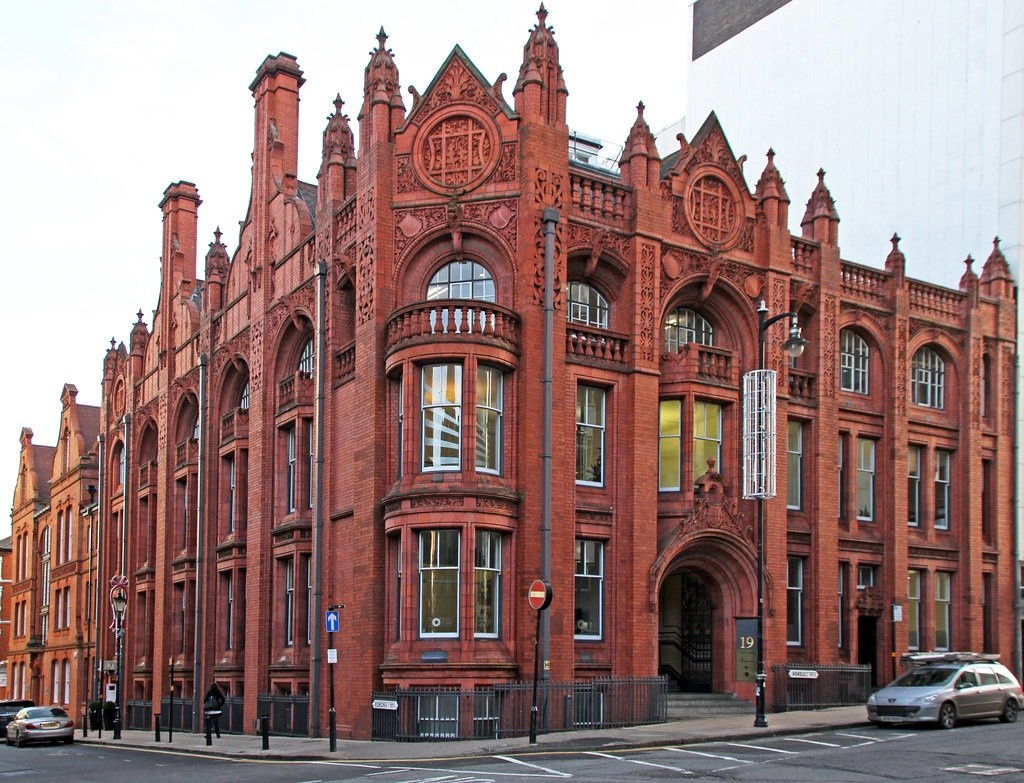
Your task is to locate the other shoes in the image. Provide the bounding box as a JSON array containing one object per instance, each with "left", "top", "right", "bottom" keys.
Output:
[
  {"left": 204, "top": 735, "right": 207, "bottom": 738},
  {"left": 217, "top": 734, "right": 220, "bottom": 738}
]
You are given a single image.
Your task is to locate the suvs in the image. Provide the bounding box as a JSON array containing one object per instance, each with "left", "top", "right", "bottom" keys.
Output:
[{"left": 0, "top": 699, "right": 35, "bottom": 739}]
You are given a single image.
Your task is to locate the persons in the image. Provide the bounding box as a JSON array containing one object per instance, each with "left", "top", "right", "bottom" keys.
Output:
[{"left": 204, "top": 683, "right": 226, "bottom": 739}]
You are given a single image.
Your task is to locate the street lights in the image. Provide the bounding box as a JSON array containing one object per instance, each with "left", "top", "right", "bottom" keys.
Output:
[{"left": 754, "top": 299, "right": 811, "bottom": 727}]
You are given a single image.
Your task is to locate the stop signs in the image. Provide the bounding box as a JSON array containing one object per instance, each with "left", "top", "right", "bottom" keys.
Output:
[{"left": 527, "top": 579, "right": 552, "bottom": 611}]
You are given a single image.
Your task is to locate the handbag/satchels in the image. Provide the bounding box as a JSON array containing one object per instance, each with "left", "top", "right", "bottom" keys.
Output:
[{"left": 204, "top": 696, "right": 218, "bottom": 710}]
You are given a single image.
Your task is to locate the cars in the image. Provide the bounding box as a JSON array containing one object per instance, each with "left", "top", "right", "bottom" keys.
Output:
[
  {"left": 867, "top": 660, "right": 1024, "bottom": 729},
  {"left": 6, "top": 706, "right": 74, "bottom": 748}
]
